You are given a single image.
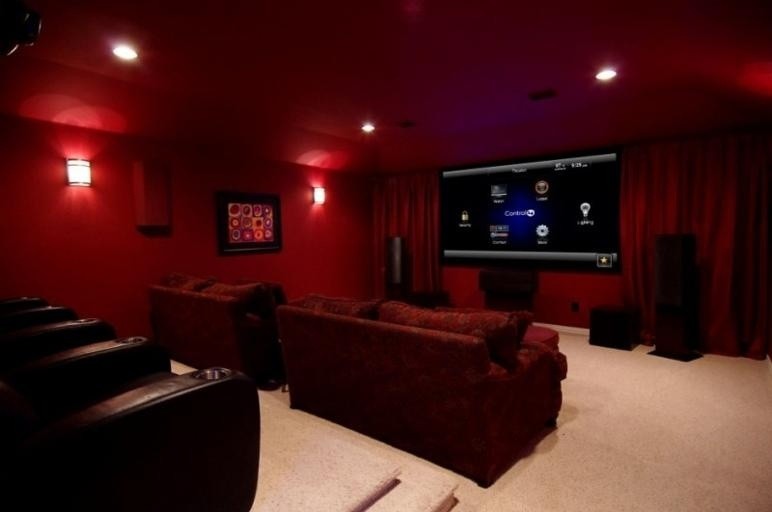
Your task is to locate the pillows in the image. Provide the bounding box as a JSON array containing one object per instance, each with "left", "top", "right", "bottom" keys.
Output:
[
  {"left": 155, "top": 274, "right": 282, "bottom": 313},
  {"left": 309, "top": 292, "right": 542, "bottom": 360}
]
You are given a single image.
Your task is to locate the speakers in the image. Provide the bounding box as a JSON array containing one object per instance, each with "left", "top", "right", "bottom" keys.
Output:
[
  {"left": 589, "top": 305, "right": 641, "bottom": 350},
  {"left": 655, "top": 233, "right": 700, "bottom": 358},
  {"left": 386, "top": 236, "right": 411, "bottom": 299},
  {"left": 407, "top": 292, "right": 449, "bottom": 309}
]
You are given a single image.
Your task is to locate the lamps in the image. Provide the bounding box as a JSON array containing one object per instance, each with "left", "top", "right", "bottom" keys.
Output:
[
  {"left": 311, "top": 185, "right": 326, "bottom": 206},
  {"left": 65, "top": 157, "right": 93, "bottom": 188}
]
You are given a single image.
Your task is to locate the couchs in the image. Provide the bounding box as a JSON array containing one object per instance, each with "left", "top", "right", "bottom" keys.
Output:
[
  {"left": 148, "top": 270, "right": 288, "bottom": 391},
  {"left": 275, "top": 289, "right": 576, "bottom": 490}
]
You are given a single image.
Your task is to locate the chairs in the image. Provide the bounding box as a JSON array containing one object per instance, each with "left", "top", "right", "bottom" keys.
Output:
[{"left": 0, "top": 294, "right": 269, "bottom": 512}]
